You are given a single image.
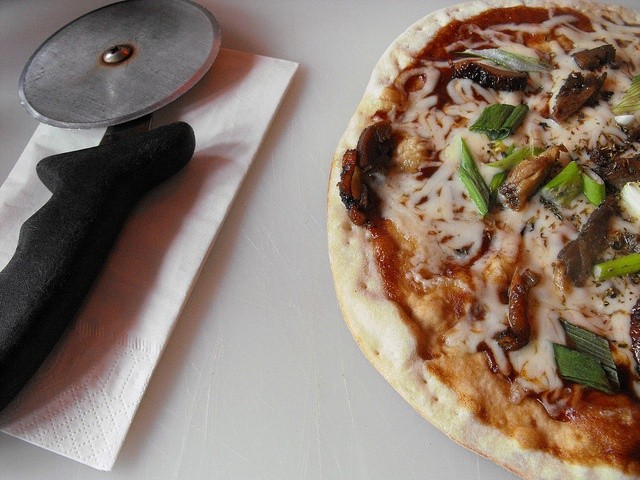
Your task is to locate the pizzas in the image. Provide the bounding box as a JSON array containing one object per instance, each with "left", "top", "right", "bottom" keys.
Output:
[{"left": 326, "top": 0, "right": 640, "bottom": 480}]
